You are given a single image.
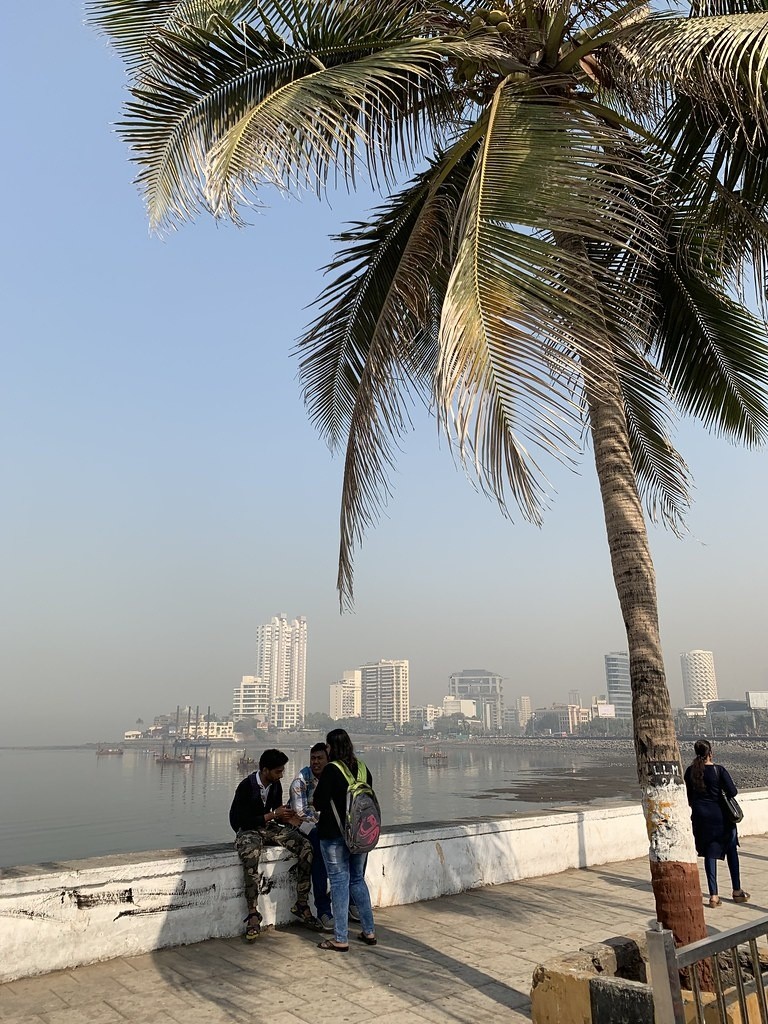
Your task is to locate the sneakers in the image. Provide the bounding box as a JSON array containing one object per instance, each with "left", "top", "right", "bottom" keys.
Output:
[
  {"left": 317, "top": 907, "right": 334, "bottom": 930},
  {"left": 348, "top": 903, "right": 361, "bottom": 923}
]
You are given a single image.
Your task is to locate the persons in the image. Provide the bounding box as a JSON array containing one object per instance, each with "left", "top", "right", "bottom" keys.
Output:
[
  {"left": 684, "top": 739, "right": 750, "bottom": 908},
  {"left": 286, "top": 743, "right": 361, "bottom": 930},
  {"left": 430, "top": 748, "right": 448, "bottom": 758},
  {"left": 313, "top": 729, "right": 377, "bottom": 952},
  {"left": 229, "top": 749, "right": 324, "bottom": 945}
]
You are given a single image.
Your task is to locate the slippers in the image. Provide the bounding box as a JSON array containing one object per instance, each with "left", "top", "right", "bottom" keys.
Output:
[
  {"left": 358, "top": 932, "right": 376, "bottom": 945},
  {"left": 318, "top": 940, "right": 348, "bottom": 952}
]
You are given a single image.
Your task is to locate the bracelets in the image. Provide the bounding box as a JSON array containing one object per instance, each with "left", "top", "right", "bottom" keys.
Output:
[{"left": 273, "top": 809, "right": 279, "bottom": 820}]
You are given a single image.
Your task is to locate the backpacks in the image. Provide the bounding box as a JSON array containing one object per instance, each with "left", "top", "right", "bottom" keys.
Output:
[{"left": 325, "top": 759, "right": 381, "bottom": 854}]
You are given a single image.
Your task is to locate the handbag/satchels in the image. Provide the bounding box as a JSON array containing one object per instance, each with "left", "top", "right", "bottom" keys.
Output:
[{"left": 714, "top": 764, "right": 744, "bottom": 823}]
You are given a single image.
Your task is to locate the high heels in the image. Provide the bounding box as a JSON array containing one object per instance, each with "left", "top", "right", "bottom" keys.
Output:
[
  {"left": 709, "top": 897, "right": 722, "bottom": 908},
  {"left": 733, "top": 890, "right": 750, "bottom": 903}
]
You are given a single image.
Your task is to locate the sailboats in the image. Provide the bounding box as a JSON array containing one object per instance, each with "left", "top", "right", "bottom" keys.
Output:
[{"left": 170, "top": 704, "right": 212, "bottom": 747}]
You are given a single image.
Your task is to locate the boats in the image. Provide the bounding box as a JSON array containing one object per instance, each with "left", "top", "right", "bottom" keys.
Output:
[
  {"left": 354, "top": 739, "right": 450, "bottom": 765},
  {"left": 154, "top": 746, "right": 199, "bottom": 761},
  {"left": 95, "top": 746, "right": 126, "bottom": 755},
  {"left": 236, "top": 747, "right": 260, "bottom": 772},
  {"left": 290, "top": 748, "right": 298, "bottom": 752}
]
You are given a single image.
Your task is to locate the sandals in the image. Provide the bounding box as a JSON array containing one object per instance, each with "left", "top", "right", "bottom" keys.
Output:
[
  {"left": 243, "top": 910, "right": 262, "bottom": 939},
  {"left": 290, "top": 902, "right": 323, "bottom": 931}
]
